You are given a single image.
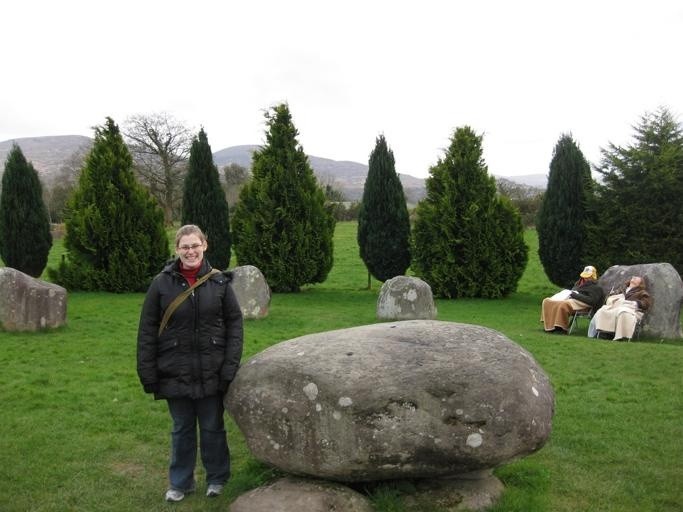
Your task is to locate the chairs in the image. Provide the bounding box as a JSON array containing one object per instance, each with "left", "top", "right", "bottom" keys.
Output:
[{"left": 540, "top": 279, "right": 646, "bottom": 343}]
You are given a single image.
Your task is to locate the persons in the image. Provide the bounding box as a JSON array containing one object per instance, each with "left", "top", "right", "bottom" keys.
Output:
[
  {"left": 594, "top": 275, "right": 652, "bottom": 342},
  {"left": 538, "top": 264, "right": 604, "bottom": 335},
  {"left": 133, "top": 225, "right": 244, "bottom": 502}
]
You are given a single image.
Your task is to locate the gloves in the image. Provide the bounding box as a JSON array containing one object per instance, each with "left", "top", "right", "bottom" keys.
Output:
[
  {"left": 143, "top": 384, "right": 156, "bottom": 393},
  {"left": 218, "top": 380, "right": 229, "bottom": 392}
]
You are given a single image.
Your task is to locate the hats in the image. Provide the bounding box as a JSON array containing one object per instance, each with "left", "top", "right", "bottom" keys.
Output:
[{"left": 580, "top": 265, "right": 597, "bottom": 277}]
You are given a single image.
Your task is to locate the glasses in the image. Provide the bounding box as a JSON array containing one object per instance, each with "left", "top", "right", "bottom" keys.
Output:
[{"left": 179, "top": 245, "right": 201, "bottom": 251}]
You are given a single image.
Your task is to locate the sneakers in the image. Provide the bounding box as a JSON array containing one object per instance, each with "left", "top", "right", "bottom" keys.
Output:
[
  {"left": 207, "top": 484, "right": 225, "bottom": 497},
  {"left": 165, "top": 480, "right": 196, "bottom": 501}
]
